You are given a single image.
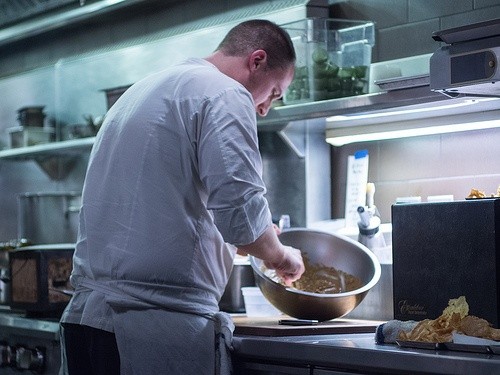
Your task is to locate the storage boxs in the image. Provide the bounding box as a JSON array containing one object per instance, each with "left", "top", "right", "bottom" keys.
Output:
[{"left": 277, "top": 17, "right": 377, "bottom": 105}]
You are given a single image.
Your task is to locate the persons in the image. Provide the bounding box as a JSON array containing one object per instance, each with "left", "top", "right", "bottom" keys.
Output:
[{"left": 59, "top": 19, "right": 306, "bottom": 375}]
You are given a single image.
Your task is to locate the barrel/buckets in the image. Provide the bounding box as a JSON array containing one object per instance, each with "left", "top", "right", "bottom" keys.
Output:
[{"left": 16, "top": 192, "right": 82, "bottom": 244}]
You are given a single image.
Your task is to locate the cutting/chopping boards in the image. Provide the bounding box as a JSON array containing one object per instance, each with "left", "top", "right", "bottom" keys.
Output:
[{"left": 229, "top": 315, "right": 388, "bottom": 335}]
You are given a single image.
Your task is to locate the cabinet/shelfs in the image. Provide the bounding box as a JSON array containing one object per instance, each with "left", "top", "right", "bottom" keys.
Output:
[{"left": 0, "top": 0, "right": 331, "bottom": 229}]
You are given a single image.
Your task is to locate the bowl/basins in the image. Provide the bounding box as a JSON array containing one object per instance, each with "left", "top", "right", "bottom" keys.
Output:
[{"left": 248, "top": 228, "right": 382, "bottom": 322}]
